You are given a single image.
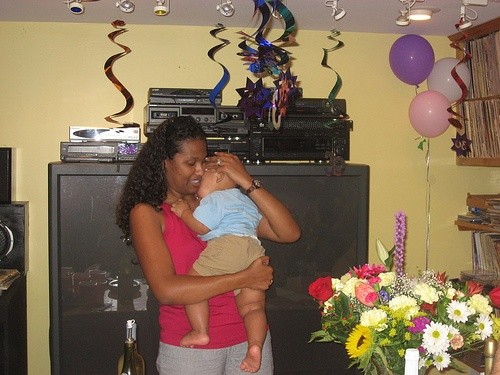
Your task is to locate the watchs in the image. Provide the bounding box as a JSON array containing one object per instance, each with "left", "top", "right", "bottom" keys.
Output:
[{"left": 245, "top": 179, "right": 263, "bottom": 196}]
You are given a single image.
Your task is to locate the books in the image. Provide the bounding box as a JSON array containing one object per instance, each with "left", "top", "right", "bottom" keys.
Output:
[
  {"left": 474, "top": 232, "right": 500, "bottom": 278},
  {"left": 0, "top": 268, "right": 21, "bottom": 295},
  {"left": 457, "top": 198, "right": 500, "bottom": 229},
  {"left": 458, "top": 31, "right": 500, "bottom": 157}
]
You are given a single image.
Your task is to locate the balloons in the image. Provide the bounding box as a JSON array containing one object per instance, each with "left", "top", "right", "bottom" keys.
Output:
[
  {"left": 388, "top": 34, "right": 435, "bottom": 85},
  {"left": 409, "top": 90, "right": 452, "bottom": 138},
  {"left": 427, "top": 57, "right": 472, "bottom": 104}
]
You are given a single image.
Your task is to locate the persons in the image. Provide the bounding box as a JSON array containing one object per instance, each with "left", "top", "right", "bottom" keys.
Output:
[
  {"left": 116, "top": 115, "right": 302, "bottom": 375},
  {"left": 171, "top": 160, "right": 268, "bottom": 372}
]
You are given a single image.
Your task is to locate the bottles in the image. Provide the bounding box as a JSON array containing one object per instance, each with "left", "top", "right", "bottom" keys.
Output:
[{"left": 117, "top": 320, "right": 145, "bottom": 375}]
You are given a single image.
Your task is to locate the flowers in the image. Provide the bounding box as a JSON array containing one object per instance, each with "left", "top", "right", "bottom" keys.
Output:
[{"left": 308, "top": 239, "right": 500, "bottom": 375}]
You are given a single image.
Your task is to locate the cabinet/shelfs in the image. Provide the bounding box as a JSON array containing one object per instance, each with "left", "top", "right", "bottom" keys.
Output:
[
  {"left": 454, "top": 221, "right": 500, "bottom": 288},
  {"left": 455, "top": 38, "right": 500, "bottom": 167},
  {"left": 49, "top": 163, "right": 369, "bottom": 375}
]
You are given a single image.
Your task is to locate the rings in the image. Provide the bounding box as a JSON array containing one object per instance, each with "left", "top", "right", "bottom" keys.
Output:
[{"left": 269, "top": 279, "right": 272, "bottom": 284}]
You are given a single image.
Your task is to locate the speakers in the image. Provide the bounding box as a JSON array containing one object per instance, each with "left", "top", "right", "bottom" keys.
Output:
[{"left": 0, "top": 203, "right": 29, "bottom": 275}]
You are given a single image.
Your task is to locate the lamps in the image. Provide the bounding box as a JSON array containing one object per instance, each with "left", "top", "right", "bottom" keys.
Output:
[
  {"left": 407, "top": 9, "right": 433, "bottom": 20},
  {"left": 67, "top": 0, "right": 84, "bottom": 14},
  {"left": 115, "top": 0, "right": 135, "bottom": 13},
  {"left": 395, "top": 8, "right": 408, "bottom": 25},
  {"left": 332, "top": 0, "right": 346, "bottom": 20},
  {"left": 216, "top": 0, "right": 234, "bottom": 16},
  {"left": 458, "top": 0, "right": 472, "bottom": 30},
  {"left": 154, "top": 0, "right": 170, "bottom": 15}
]
